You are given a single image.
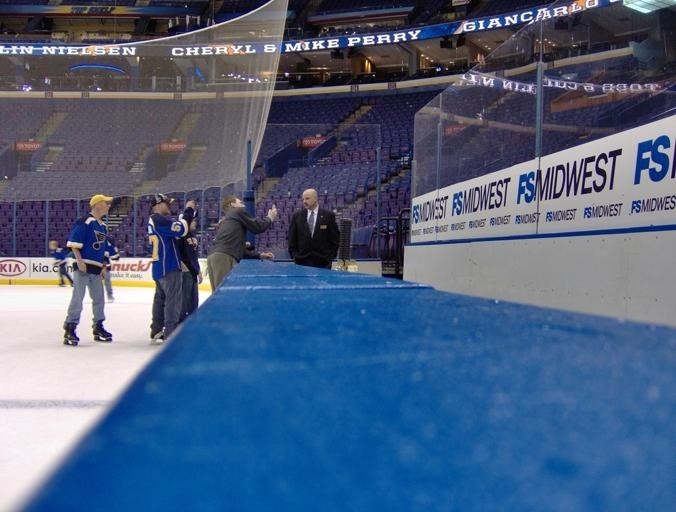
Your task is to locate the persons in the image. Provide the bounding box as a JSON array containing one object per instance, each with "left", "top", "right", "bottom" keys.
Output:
[
  {"left": 180, "top": 209, "right": 201, "bottom": 326},
  {"left": 48, "top": 241, "right": 75, "bottom": 287},
  {"left": 102, "top": 236, "right": 121, "bottom": 302},
  {"left": 146, "top": 193, "right": 196, "bottom": 344},
  {"left": 205, "top": 199, "right": 278, "bottom": 292},
  {"left": 62, "top": 194, "right": 116, "bottom": 347},
  {"left": 287, "top": 187, "right": 340, "bottom": 271}
]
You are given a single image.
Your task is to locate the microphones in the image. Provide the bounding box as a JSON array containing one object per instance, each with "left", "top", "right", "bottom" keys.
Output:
[{"left": 271, "top": 204, "right": 275, "bottom": 230}]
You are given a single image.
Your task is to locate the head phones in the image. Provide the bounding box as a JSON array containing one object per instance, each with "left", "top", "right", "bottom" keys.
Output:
[{"left": 153, "top": 191, "right": 162, "bottom": 204}]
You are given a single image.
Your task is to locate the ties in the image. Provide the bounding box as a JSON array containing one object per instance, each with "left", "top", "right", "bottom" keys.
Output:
[{"left": 308, "top": 210, "right": 314, "bottom": 234}]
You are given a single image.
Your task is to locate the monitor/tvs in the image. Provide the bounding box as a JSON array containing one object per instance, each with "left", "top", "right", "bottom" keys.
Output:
[{"left": 330, "top": 20, "right": 569, "bottom": 60}]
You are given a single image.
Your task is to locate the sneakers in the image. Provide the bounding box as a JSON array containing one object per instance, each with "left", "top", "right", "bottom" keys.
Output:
[
  {"left": 150, "top": 331, "right": 164, "bottom": 339},
  {"left": 93, "top": 328, "right": 112, "bottom": 339},
  {"left": 63, "top": 329, "right": 79, "bottom": 342}
]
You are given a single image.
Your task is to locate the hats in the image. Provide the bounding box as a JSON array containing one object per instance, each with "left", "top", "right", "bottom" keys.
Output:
[
  {"left": 151, "top": 192, "right": 170, "bottom": 206},
  {"left": 89, "top": 193, "right": 113, "bottom": 205}
]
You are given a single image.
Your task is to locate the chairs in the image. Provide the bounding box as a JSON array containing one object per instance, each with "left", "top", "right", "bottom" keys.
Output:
[{"left": 0, "top": 50, "right": 670, "bottom": 266}]
